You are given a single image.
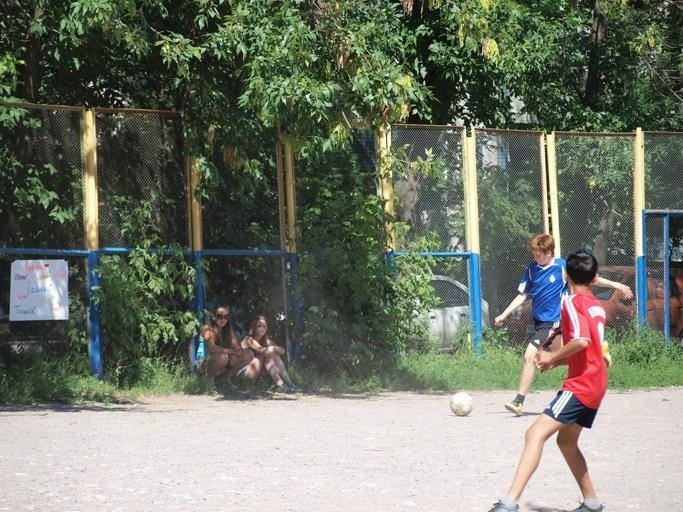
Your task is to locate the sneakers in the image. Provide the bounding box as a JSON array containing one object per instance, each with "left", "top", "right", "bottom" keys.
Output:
[
  {"left": 505, "top": 399, "right": 524, "bottom": 417},
  {"left": 571, "top": 501, "right": 605, "bottom": 512},
  {"left": 485, "top": 500, "right": 521, "bottom": 512},
  {"left": 278, "top": 382, "right": 306, "bottom": 393}
]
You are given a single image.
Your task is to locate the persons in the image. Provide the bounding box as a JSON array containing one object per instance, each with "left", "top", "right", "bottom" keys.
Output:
[
  {"left": 485, "top": 250, "right": 608, "bottom": 512},
  {"left": 233, "top": 313, "right": 305, "bottom": 394},
  {"left": 196, "top": 302, "right": 253, "bottom": 391},
  {"left": 494, "top": 234, "right": 634, "bottom": 415}
]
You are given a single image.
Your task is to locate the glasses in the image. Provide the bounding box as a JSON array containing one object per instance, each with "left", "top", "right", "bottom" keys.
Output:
[{"left": 216, "top": 314, "right": 230, "bottom": 320}]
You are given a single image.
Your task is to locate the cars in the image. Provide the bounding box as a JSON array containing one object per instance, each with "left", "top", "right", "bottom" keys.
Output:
[
  {"left": 507, "top": 266, "right": 682, "bottom": 344},
  {"left": 398, "top": 275, "right": 490, "bottom": 354}
]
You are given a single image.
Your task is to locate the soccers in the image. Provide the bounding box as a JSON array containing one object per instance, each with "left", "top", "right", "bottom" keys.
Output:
[{"left": 451, "top": 392, "right": 474, "bottom": 416}]
